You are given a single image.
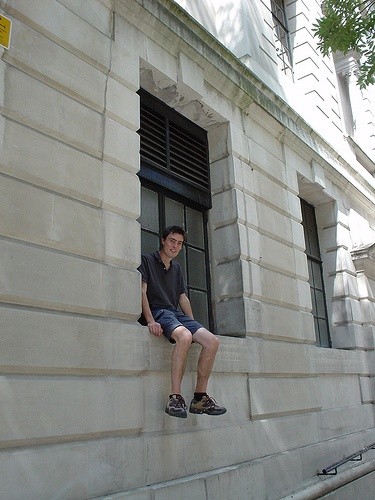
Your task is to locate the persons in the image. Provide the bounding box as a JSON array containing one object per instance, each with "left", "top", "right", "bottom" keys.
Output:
[{"left": 141, "top": 225, "right": 227, "bottom": 418}]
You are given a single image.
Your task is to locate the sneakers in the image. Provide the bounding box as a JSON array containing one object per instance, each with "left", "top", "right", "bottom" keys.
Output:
[
  {"left": 165, "top": 395, "right": 187, "bottom": 419},
  {"left": 189, "top": 394, "right": 228, "bottom": 415}
]
